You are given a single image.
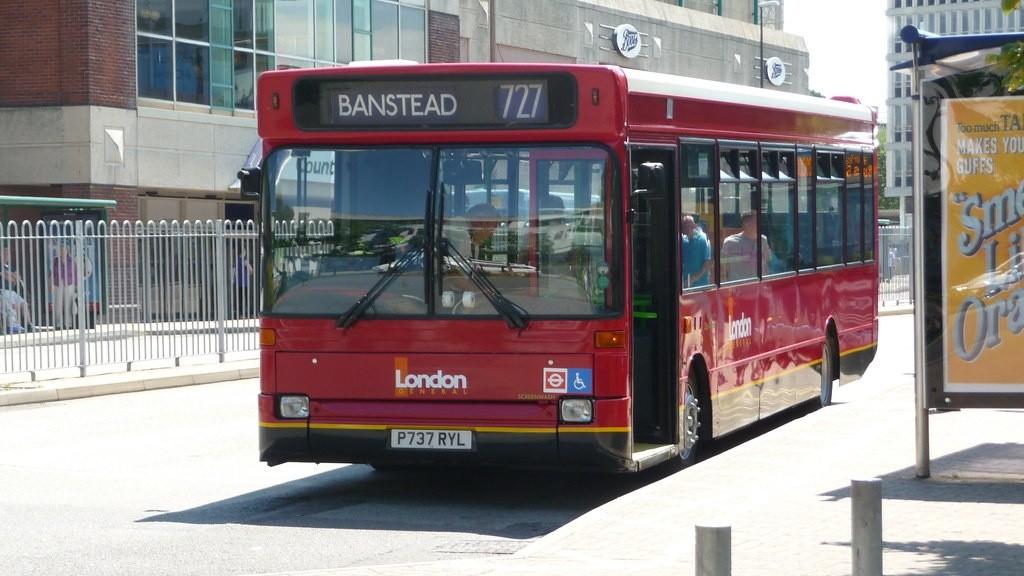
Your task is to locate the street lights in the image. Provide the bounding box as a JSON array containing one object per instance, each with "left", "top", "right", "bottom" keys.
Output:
[{"left": 758, "top": 1, "right": 779, "bottom": 88}]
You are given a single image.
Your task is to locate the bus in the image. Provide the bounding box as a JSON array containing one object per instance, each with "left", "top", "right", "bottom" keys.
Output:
[{"left": 238, "top": 59, "right": 878, "bottom": 479}]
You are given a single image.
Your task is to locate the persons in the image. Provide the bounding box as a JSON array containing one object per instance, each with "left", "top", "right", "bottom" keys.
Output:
[
  {"left": 681, "top": 215, "right": 712, "bottom": 289},
  {"left": 421, "top": 199, "right": 502, "bottom": 262},
  {"left": 0, "top": 246, "right": 36, "bottom": 333},
  {"left": 230, "top": 247, "right": 259, "bottom": 319},
  {"left": 518, "top": 192, "right": 576, "bottom": 278},
  {"left": 711, "top": 210, "right": 771, "bottom": 282},
  {"left": 47, "top": 243, "right": 80, "bottom": 330}
]
